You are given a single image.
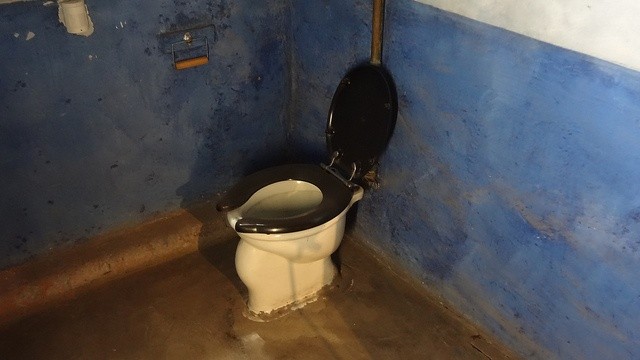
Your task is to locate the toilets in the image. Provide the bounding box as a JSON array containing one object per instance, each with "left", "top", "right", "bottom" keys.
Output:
[{"left": 214, "top": 58, "right": 400, "bottom": 323}]
